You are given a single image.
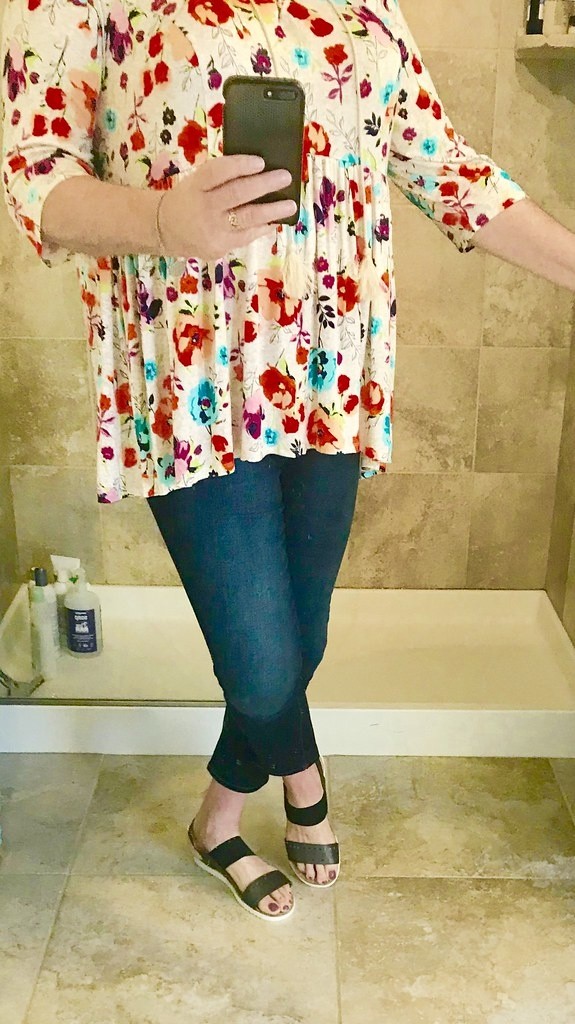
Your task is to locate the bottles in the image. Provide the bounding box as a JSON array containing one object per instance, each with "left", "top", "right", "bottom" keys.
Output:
[{"left": 32, "top": 566, "right": 105, "bottom": 682}]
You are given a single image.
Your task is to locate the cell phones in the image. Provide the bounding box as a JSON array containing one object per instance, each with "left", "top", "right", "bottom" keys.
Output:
[{"left": 221, "top": 76, "right": 305, "bottom": 227}]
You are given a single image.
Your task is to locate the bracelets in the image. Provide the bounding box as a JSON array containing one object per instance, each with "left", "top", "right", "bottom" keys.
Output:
[{"left": 155, "top": 192, "right": 169, "bottom": 251}]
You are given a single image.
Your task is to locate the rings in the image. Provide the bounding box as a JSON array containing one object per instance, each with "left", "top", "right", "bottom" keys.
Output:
[{"left": 228, "top": 208, "right": 240, "bottom": 230}]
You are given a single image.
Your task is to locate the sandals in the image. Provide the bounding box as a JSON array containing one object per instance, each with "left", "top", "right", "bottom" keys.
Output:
[
  {"left": 188, "top": 816, "right": 296, "bottom": 920},
  {"left": 283, "top": 752, "right": 340, "bottom": 888}
]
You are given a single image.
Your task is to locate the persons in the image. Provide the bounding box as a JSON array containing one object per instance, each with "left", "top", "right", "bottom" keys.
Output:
[{"left": 0, "top": 0, "right": 574, "bottom": 919}]
